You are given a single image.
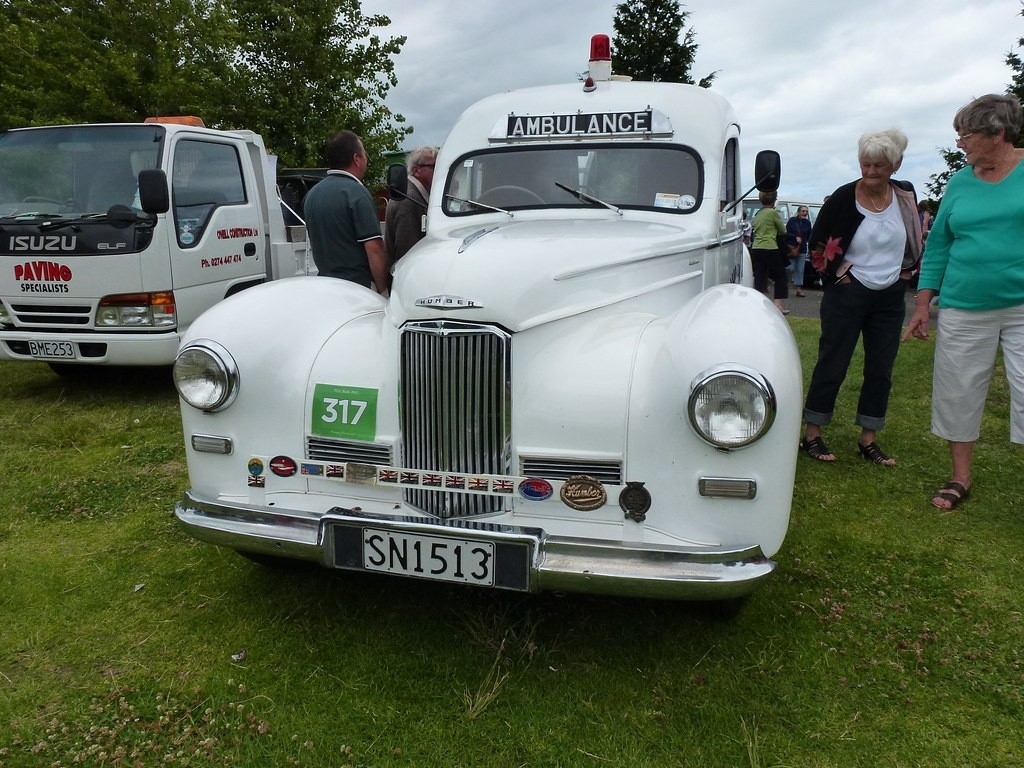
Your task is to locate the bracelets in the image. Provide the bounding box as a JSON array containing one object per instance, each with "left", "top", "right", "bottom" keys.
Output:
[{"left": 834, "top": 275, "right": 848, "bottom": 285}]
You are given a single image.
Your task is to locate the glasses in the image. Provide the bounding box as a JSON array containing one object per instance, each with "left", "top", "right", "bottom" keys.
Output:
[{"left": 955, "top": 132, "right": 975, "bottom": 143}]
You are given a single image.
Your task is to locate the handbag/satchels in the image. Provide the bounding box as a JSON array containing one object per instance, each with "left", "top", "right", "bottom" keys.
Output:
[
  {"left": 777, "top": 233, "right": 791, "bottom": 256},
  {"left": 788, "top": 244, "right": 799, "bottom": 257}
]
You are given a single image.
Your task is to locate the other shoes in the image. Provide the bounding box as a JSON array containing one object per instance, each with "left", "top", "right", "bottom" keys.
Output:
[
  {"left": 796, "top": 292, "right": 806, "bottom": 297},
  {"left": 779, "top": 308, "right": 790, "bottom": 315}
]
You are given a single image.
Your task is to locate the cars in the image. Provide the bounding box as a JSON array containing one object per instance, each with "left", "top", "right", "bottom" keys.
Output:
[
  {"left": 743, "top": 195, "right": 824, "bottom": 233},
  {"left": 168, "top": 33, "right": 807, "bottom": 633}
]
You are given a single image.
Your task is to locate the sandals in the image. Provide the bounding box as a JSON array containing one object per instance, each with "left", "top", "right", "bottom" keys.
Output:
[
  {"left": 859, "top": 441, "right": 897, "bottom": 467},
  {"left": 800, "top": 436, "right": 837, "bottom": 462}
]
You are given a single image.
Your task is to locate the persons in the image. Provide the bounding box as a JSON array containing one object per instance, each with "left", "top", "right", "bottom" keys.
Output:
[
  {"left": 899, "top": 91, "right": 1024, "bottom": 511},
  {"left": 743, "top": 210, "right": 753, "bottom": 256},
  {"left": 785, "top": 205, "right": 812, "bottom": 298},
  {"left": 808, "top": 125, "right": 924, "bottom": 466},
  {"left": 303, "top": 129, "right": 391, "bottom": 300},
  {"left": 821, "top": 188, "right": 944, "bottom": 299},
  {"left": 749, "top": 190, "right": 791, "bottom": 315},
  {"left": 383, "top": 144, "right": 440, "bottom": 300}
]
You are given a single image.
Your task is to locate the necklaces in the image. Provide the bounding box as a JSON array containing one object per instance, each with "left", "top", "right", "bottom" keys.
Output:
[{"left": 864, "top": 183, "right": 889, "bottom": 211}]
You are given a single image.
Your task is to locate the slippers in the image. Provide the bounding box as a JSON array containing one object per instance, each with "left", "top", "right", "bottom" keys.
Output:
[{"left": 932, "top": 477, "right": 974, "bottom": 511}]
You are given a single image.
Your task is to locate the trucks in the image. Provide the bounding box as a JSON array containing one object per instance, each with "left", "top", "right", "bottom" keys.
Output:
[{"left": 1, "top": 115, "right": 386, "bottom": 386}]
